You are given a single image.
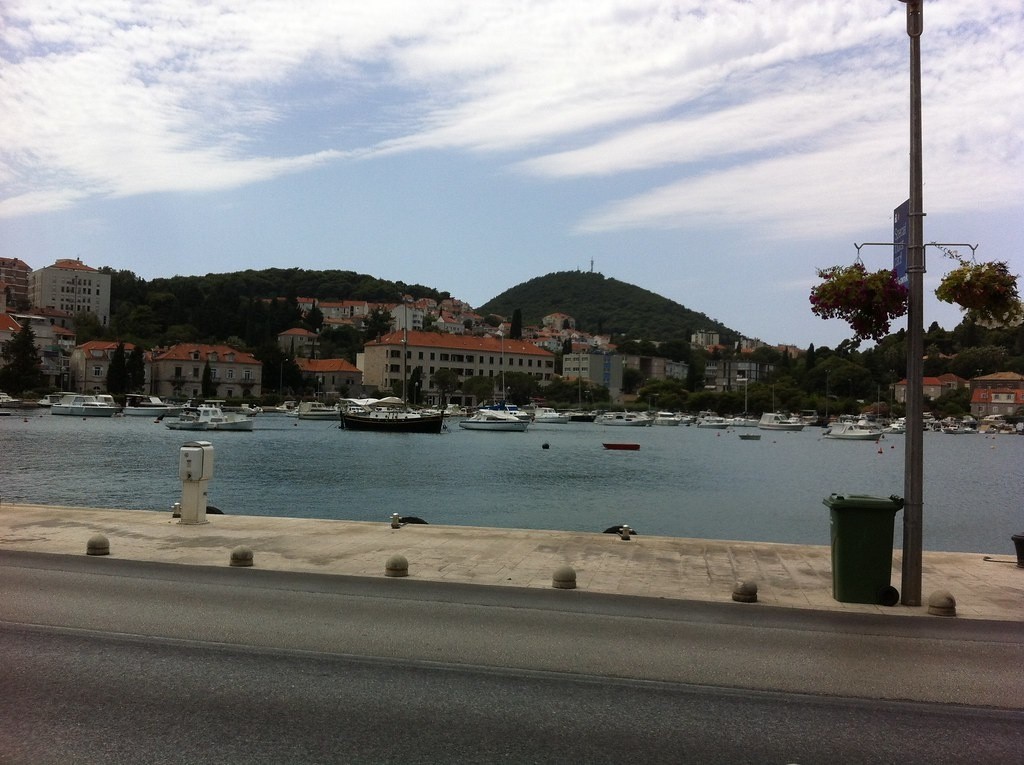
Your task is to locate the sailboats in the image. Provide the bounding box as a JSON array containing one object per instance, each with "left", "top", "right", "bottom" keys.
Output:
[
  {"left": 726, "top": 361, "right": 760, "bottom": 427},
  {"left": 563, "top": 338, "right": 598, "bottom": 422},
  {"left": 339, "top": 297, "right": 445, "bottom": 434}
]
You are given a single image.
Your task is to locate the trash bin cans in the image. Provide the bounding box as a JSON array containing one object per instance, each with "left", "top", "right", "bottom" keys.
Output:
[{"left": 822, "top": 491, "right": 904, "bottom": 607}]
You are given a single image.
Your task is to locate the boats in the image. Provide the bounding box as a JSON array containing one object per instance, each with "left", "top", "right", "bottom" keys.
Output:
[
  {"left": 459, "top": 409, "right": 530, "bottom": 433},
  {"left": 821, "top": 420, "right": 883, "bottom": 441},
  {"left": 51, "top": 395, "right": 123, "bottom": 417},
  {"left": 38, "top": 391, "right": 116, "bottom": 408},
  {"left": 757, "top": 413, "right": 807, "bottom": 431},
  {"left": 741, "top": 434, "right": 761, "bottom": 440},
  {"left": 123, "top": 394, "right": 179, "bottom": 416},
  {"left": 166, "top": 407, "right": 254, "bottom": 431},
  {"left": 589, "top": 410, "right": 730, "bottom": 429},
  {"left": 0, "top": 392, "right": 23, "bottom": 408},
  {"left": 602, "top": 443, "right": 640, "bottom": 451},
  {"left": 275, "top": 400, "right": 570, "bottom": 424},
  {"left": 884, "top": 413, "right": 1024, "bottom": 435},
  {"left": 181, "top": 398, "right": 257, "bottom": 417}
]
